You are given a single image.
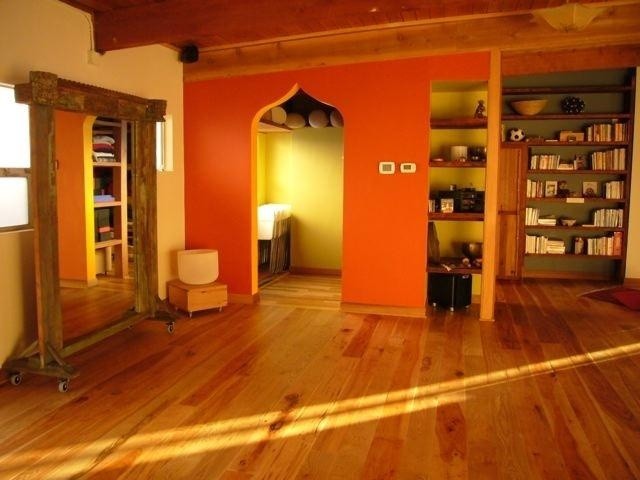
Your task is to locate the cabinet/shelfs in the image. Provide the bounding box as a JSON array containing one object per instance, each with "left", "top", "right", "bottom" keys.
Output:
[
  {"left": 500, "top": 114, "right": 634, "bottom": 288},
  {"left": 428, "top": 116, "right": 486, "bottom": 275},
  {"left": 93, "top": 120, "right": 121, "bottom": 251},
  {"left": 166, "top": 278, "right": 229, "bottom": 319}
]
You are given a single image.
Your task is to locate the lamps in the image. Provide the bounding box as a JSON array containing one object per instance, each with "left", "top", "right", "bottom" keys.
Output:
[{"left": 530, "top": 1, "right": 609, "bottom": 34}]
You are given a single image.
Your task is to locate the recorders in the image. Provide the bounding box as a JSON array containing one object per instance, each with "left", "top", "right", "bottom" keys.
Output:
[{"left": 439, "top": 188, "right": 485, "bottom": 212}]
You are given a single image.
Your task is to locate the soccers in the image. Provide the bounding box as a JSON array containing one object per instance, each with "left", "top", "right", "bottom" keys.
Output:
[{"left": 508, "top": 128, "right": 525, "bottom": 142}]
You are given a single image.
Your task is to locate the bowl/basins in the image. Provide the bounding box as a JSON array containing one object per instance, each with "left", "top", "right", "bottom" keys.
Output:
[
  {"left": 462, "top": 242, "right": 482, "bottom": 257},
  {"left": 510, "top": 99, "right": 549, "bottom": 115}
]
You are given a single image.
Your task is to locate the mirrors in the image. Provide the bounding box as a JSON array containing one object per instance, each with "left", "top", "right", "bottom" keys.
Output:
[{"left": 3, "top": 70, "right": 178, "bottom": 393}]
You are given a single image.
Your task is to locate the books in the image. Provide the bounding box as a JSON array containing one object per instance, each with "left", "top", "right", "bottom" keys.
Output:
[{"left": 525, "top": 121, "right": 627, "bottom": 256}]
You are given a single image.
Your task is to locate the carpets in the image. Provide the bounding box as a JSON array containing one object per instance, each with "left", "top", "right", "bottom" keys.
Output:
[{"left": 581, "top": 284, "right": 640, "bottom": 313}]
